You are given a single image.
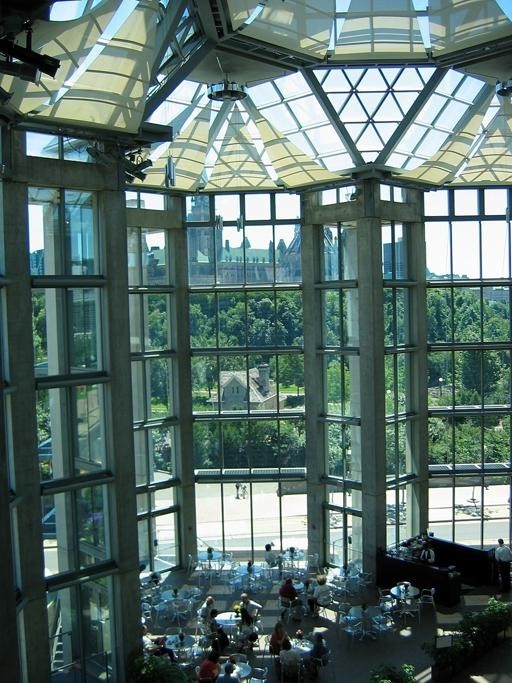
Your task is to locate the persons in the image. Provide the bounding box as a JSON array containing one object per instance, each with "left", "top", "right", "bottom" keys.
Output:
[
  {"left": 494, "top": 538, "right": 511, "bottom": 592},
  {"left": 419, "top": 542, "right": 436, "bottom": 564},
  {"left": 141, "top": 543, "right": 333, "bottom": 682}
]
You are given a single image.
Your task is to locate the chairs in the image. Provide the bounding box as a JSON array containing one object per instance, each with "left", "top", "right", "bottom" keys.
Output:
[
  {"left": 136, "top": 530, "right": 436, "bottom": 683},
  {"left": 454, "top": 494, "right": 497, "bottom": 519},
  {"left": 385, "top": 500, "right": 410, "bottom": 523}
]
